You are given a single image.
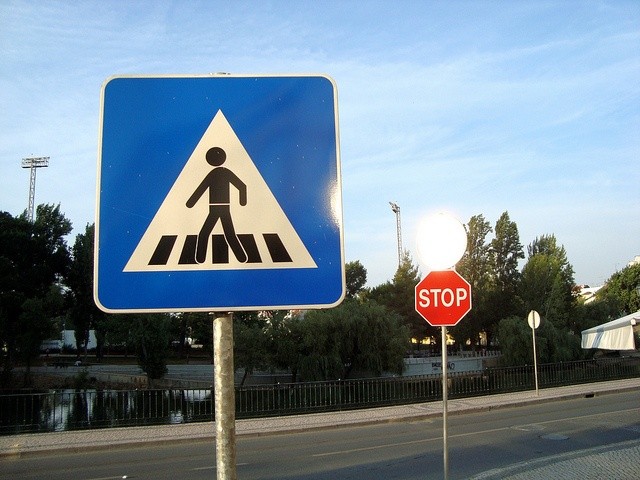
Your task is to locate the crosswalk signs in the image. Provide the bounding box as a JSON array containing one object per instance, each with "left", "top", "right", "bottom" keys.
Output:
[{"left": 94, "top": 73, "right": 346, "bottom": 314}]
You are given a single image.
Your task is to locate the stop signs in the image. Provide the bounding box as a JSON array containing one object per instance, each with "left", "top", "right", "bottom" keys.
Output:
[{"left": 415, "top": 270, "right": 471, "bottom": 326}]
